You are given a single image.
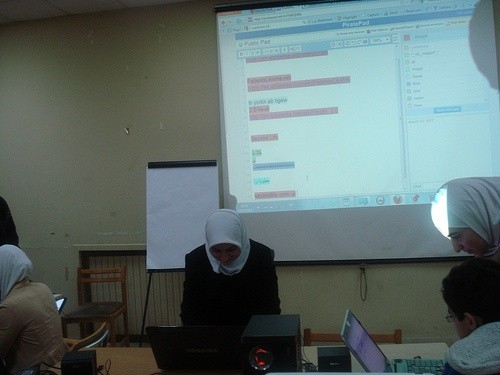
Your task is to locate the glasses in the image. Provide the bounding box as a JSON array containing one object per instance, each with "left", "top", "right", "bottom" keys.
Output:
[{"left": 446, "top": 312, "right": 458, "bottom": 323}]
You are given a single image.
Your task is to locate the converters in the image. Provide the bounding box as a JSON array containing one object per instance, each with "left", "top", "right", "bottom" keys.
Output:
[{"left": 20, "top": 368, "right": 40, "bottom": 375}]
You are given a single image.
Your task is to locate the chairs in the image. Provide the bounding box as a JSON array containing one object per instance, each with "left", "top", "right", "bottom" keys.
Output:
[
  {"left": 60, "top": 265, "right": 130, "bottom": 348},
  {"left": 303, "top": 328, "right": 401, "bottom": 346}
]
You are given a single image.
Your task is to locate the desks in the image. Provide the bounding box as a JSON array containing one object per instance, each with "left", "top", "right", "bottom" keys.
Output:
[{"left": 49, "top": 342, "right": 453, "bottom": 375}]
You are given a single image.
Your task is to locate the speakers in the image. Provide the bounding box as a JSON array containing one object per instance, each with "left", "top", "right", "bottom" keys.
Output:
[
  {"left": 61, "top": 350, "right": 96, "bottom": 375},
  {"left": 241, "top": 314, "right": 302, "bottom": 375},
  {"left": 317, "top": 347, "right": 351, "bottom": 372}
]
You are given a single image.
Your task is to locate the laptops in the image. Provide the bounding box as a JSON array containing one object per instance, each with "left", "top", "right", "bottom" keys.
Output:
[
  {"left": 53, "top": 293, "right": 67, "bottom": 312},
  {"left": 145, "top": 326, "right": 241, "bottom": 370},
  {"left": 340, "top": 309, "right": 448, "bottom": 375}
]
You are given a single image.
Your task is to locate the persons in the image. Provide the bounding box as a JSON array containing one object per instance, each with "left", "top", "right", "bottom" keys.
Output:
[
  {"left": 439, "top": 260, "right": 500, "bottom": 375},
  {"left": 177, "top": 208, "right": 282, "bottom": 336},
  {"left": 0, "top": 245, "right": 66, "bottom": 375},
  {"left": 430, "top": 177, "right": 500, "bottom": 262}
]
